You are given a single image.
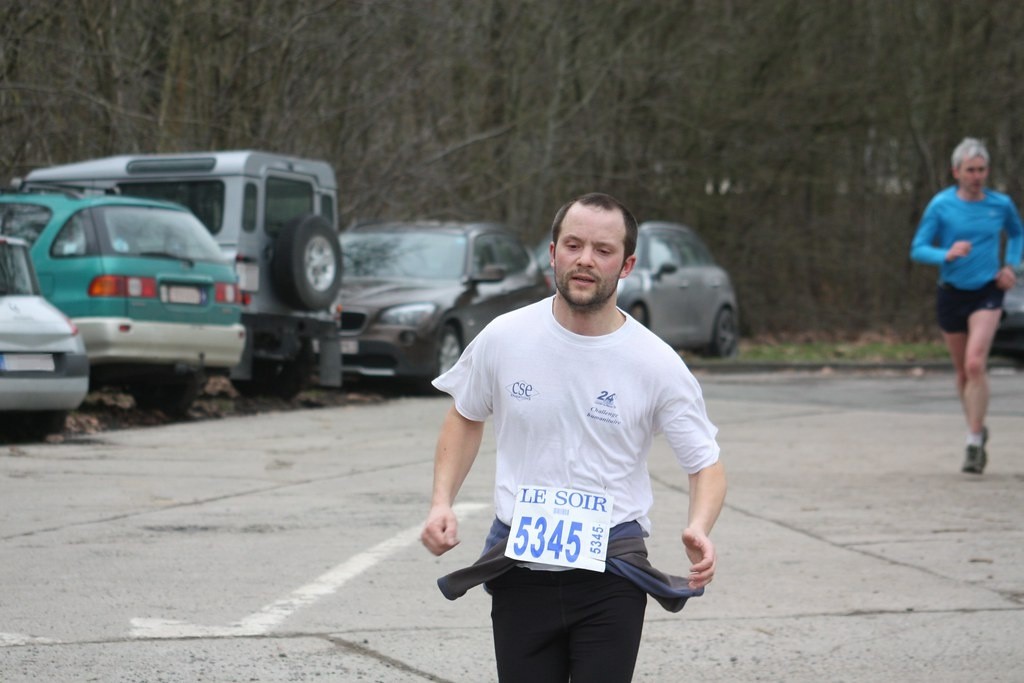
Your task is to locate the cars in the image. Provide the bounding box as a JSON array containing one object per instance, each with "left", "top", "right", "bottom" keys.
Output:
[
  {"left": 992, "top": 267, "right": 1023, "bottom": 356},
  {"left": 277, "top": 219, "right": 551, "bottom": 399},
  {"left": 0, "top": 234, "right": 90, "bottom": 440},
  {"left": 527, "top": 219, "right": 744, "bottom": 362}
]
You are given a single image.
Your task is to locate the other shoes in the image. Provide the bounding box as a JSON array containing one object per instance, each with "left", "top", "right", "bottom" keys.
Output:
[{"left": 963, "top": 447, "right": 986, "bottom": 471}]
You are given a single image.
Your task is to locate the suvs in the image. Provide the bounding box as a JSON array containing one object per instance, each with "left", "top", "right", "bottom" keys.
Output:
[{"left": 11, "top": 149, "right": 345, "bottom": 401}]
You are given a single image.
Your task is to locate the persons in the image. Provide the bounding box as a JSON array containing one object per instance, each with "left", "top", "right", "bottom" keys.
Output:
[
  {"left": 909, "top": 136, "right": 1024, "bottom": 473},
  {"left": 421, "top": 191, "right": 729, "bottom": 683}
]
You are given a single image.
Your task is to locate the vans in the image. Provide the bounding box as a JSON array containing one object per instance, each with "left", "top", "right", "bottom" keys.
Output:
[{"left": 1, "top": 179, "right": 244, "bottom": 414}]
